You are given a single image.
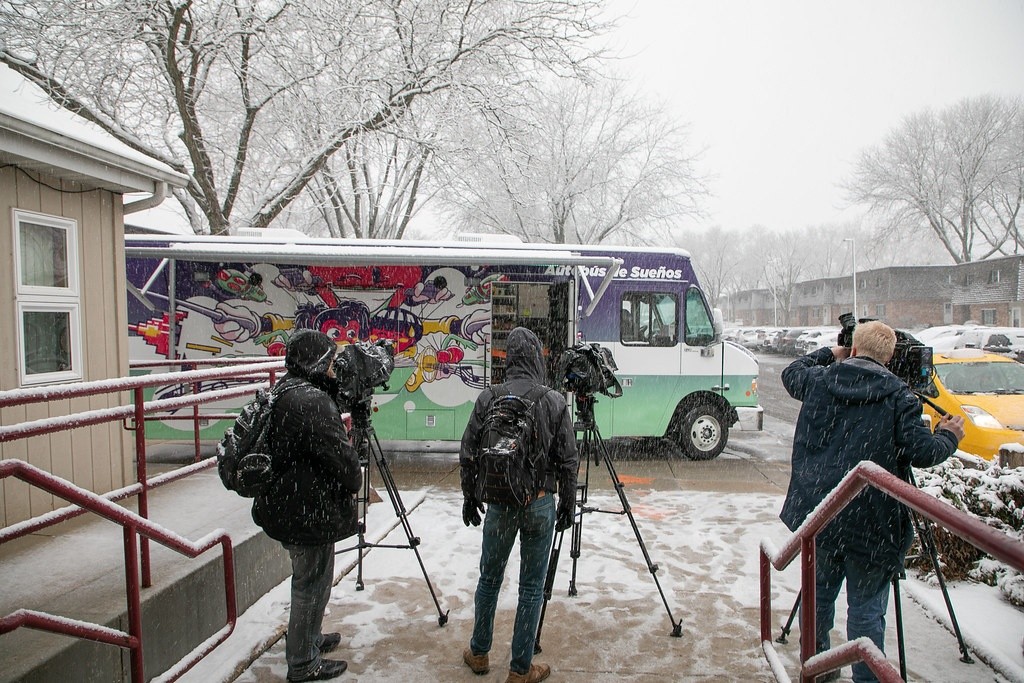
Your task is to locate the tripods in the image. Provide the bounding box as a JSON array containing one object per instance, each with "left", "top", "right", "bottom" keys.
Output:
[
  {"left": 775, "top": 467, "right": 975, "bottom": 683},
  {"left": 531, "top": 394, "right": 685, "bottom": 652},
  {"left": 334, "top": 388, "right": 450, "bottom": 627}
]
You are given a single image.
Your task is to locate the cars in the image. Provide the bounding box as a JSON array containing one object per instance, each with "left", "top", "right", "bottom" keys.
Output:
[
  {"left": 922, "top": 348, "right": 1024, "bottom": 461},
  {"left": 724, "top": 323, "right": 1024, "bottom": 371}
]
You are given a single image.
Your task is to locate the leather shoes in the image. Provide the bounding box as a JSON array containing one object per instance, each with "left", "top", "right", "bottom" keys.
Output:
[
  {"left": 504, "top": 662, "right": 551, "bottom": 683},
  {"left": 317, "top": 631, "right": 341, "bottom": 652},
  {"left": 462, "top": 646, "right": 490, "bottom": 674},
  {"left": 287, "top": 658, "right": 347, "bottom": 683}
]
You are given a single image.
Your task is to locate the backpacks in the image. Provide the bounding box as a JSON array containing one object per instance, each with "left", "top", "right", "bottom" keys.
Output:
[
  {"left": 477, "top": 382, "right": 553, "bottom": 510},
  {"left": 217, "top": 377, "right": 307, "bottom": 498}
]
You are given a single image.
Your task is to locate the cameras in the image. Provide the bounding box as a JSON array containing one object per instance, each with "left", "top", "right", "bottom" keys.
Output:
[
  {"left": 561, "top": 344, "right": 615, "bottom": 394},
  {"left": 837, "top": 312, "right": 934, "bottom": 389},
  {"left": 336, "top": 338, "right": 395, "bottom": 388}
]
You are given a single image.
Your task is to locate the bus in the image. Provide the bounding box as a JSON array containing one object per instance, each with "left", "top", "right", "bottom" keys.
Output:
[{"left": 125, "top": 228, "right": 761, "bottom": 463}]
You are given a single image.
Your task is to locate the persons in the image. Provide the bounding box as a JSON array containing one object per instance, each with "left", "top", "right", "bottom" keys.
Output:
[
  {"left": 781, "top": 319, "right": 967, "bottom": 682},
  {"left": 620, "top": 309, "right": 645, "bottom": 344},
  {"left": 458, "top": 324, "right": 582, "bottom": 683},
  {"left": 249, "top": 328, "right": 364, "bottom": 683}
]
